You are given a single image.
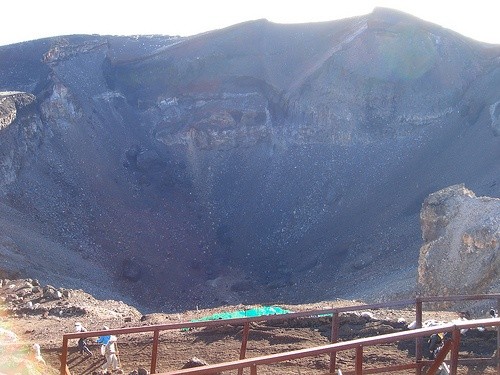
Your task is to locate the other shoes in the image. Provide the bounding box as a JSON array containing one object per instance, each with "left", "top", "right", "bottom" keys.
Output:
[
  {"left": 78, "top": 351, "right": 82, "bottom": 354},
  {"left": 101, "top": 366, "right": 104, "bottom": 370},
  {"left": 111, "top": 368, "right": 117, "bottom": 371},
  {"left": 87, "top": 354, "right": 92, "bottom": 357}
]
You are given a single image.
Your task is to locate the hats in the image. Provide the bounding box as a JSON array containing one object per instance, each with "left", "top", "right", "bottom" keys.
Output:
[{"left": 109, "top": 335, "right": 118, "bottom": 342}]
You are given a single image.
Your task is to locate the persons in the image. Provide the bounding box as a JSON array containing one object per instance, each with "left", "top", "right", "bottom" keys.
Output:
[
  {"left": 96, "top": 326, "right": 120, "bottom": 371},
  {"left": 8, "top": 343, "right": 47, "bottom": 375},
  {"left": 455, "top": 307, "right": 499, "bottom": 353},
  {"left": 75, "top": 322, "right": 93, "bottom": 357},
  {"left": 429, "top": 332, "right": 450, "bottom": 375}
]
